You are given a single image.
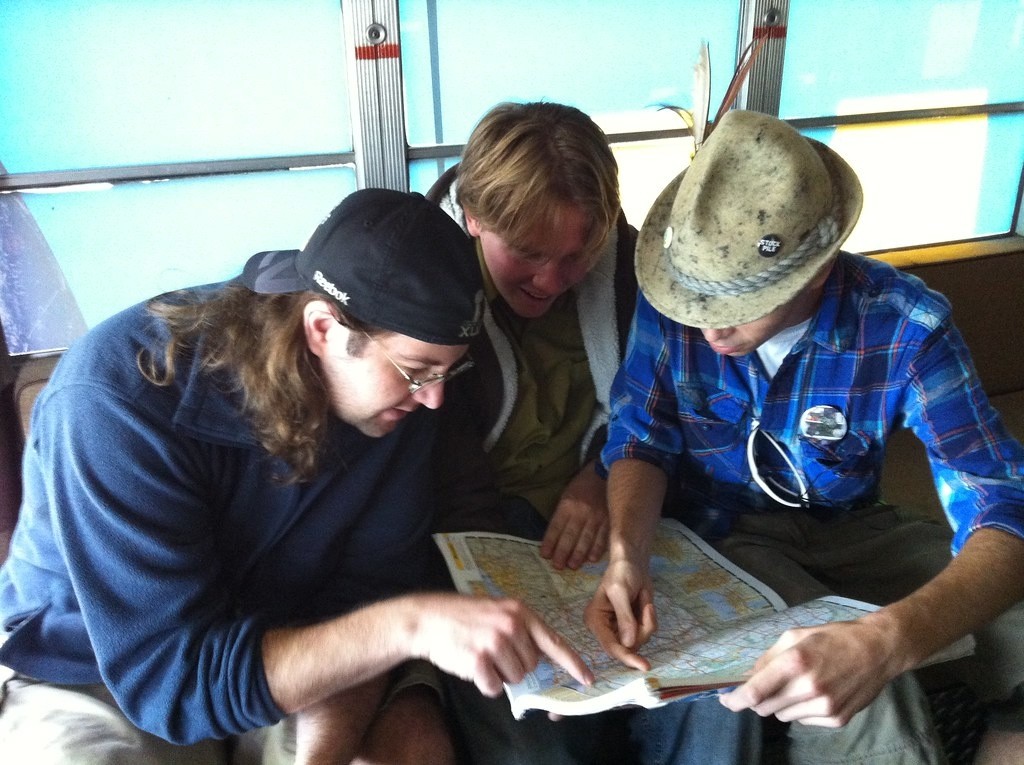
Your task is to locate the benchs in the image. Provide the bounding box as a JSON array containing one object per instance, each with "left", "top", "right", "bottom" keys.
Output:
[{"left": 13, "top": 235, "right": 1024, "bottom": 764}]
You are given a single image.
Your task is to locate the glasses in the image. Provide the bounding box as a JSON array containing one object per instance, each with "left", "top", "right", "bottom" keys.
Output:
[
  {"left": 360, "top": 328, "right": 476, "bottom": 395},
  {"left": 747, "top": 425, "right": 810, "bottom": 509}
]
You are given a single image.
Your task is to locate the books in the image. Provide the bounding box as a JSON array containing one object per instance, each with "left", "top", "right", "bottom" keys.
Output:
[{"left": 434, "top": 519, "right": 976, "bottom": 724}]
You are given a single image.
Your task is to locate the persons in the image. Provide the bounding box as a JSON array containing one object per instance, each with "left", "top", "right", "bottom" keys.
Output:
[
  {"left": 583, "top": 112, "right": 1024, "bottom": 765},
  {"left": 0, "top": 189, "right": 597, "bottom": 765},
  {"left": 422, "top": 104, "right": 766, "bottom": 765}
]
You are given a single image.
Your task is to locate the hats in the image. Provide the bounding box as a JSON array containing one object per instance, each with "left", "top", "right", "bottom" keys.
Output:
[
  {"left": 243, "top": 188, "right": 482, "bottom": 346},
  {"left": 633, "top": 108, "right": 863, "bottom": 330}
]
